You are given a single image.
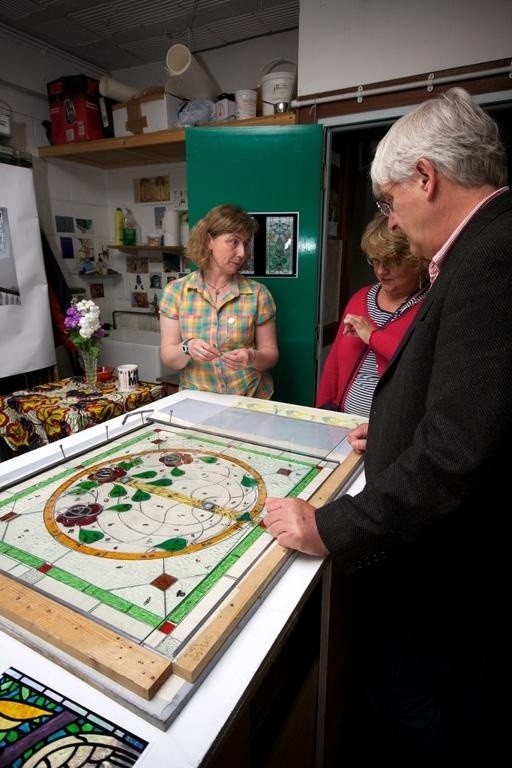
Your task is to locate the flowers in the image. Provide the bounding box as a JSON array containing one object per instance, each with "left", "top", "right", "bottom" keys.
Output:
[{"left": 63, "top": 298, "right": 107, "bottom": 393}]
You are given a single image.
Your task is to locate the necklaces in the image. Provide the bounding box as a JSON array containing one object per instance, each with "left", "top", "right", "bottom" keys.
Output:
[{"left": 203, "top": 275, "right": 231, "bottom": 300}]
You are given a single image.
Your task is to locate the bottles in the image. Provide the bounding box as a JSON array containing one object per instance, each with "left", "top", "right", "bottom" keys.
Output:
[{"left": 114, "top": 207, "right": 137, "bottom": 247}]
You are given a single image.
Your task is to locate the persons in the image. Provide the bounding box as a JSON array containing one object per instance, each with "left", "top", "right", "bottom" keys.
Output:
[
  {"left": 262, "top": 89, "right": 512, "bottom": 767},
  {"left": 317, "top": 214, "right": 426, "bottom": 417},
  {"left": 160, "top": 197, "right": 279, "bottom": 403}
]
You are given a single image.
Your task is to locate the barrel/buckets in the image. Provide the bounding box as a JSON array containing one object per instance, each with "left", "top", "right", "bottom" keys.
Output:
[{"left": 258, "top": 57, "right": 297, "bottom": 117}]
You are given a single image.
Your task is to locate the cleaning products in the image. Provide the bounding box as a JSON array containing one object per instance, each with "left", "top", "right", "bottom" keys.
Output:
[
  {"left": 115, "top": 208, "right": 124, "bottom": 246},
  {"left": 124, "top": 207, "right": 137, "bottom": 246}
]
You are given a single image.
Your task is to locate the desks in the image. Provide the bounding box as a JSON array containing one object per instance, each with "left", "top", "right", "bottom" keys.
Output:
[{"left": 1, "top": 374, "right": 372, "bottom": 765}]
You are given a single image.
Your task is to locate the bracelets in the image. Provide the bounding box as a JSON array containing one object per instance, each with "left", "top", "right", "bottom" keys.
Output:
[{"left": 249, "top": 348, "right": 256, "bottom": 362}]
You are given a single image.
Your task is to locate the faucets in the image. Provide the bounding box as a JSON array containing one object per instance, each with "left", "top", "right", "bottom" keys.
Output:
[{"left": 149, "top": 292, "right": 161, "bottom": 320}]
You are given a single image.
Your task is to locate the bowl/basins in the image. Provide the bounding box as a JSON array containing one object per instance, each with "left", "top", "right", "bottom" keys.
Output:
[{"left": 96, "top": 366, "right": 116, "bottom": 381}]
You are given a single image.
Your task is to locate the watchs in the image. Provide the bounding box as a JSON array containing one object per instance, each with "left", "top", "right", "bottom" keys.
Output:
[{"left": 183, "top": 338, "right": 195, "bottom": 356}]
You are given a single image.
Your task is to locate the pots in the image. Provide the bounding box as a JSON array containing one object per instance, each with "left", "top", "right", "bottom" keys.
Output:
[{"left": 260, "top": 99, "right": 289, "bottom": 114}]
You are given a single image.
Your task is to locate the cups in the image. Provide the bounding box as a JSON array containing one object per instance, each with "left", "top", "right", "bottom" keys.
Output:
[
  {"left": 117, "top": 364, "right": 138, "bottom": 390},
  {"left": 146, "top": 233, "right": 162, "bottom": 247},
  {"left": 163, "top": 210, "right": 180, "bottom": 247},
  {"left": 234, "top": 90, "right": 257, "bottom": 121}
]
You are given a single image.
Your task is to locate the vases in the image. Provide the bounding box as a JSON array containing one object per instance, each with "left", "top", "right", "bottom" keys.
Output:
[{"left": 80, "top": 347, "right": 99, "bottom": 394}]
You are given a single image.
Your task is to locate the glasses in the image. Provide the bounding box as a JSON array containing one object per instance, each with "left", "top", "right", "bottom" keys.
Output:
[
  {"left": 375, "top": 179, "right": 400, "bottom": 219},
  {"left": 365, "top": 254, "right": 403, "bottom": 267}
]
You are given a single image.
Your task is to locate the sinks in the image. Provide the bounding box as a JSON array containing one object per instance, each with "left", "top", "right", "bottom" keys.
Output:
[{"left": 101, "top": 326, "right": 181, "bottom": 386}]
